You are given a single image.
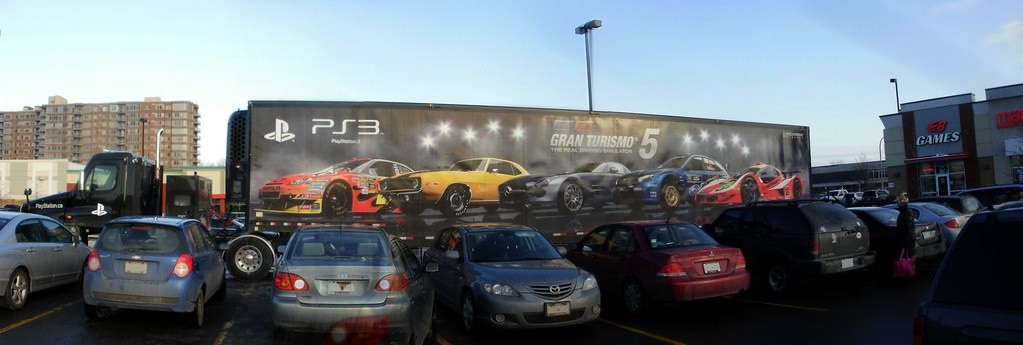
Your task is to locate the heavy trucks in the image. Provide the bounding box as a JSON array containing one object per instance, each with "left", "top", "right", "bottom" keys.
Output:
[{"left": 20, "top": 101, "right": 811, "bottom": 275}]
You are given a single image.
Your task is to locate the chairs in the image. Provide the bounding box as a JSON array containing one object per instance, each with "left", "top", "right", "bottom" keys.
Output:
[
  {"left": 469, "top": 235, "right": 480, "bottom": 260},
  {"left": 496, "top": 235, "right": 525, "bottom": 258},
  {"left": 357, "top": 243, "right": 380, "bottom": 257},
  {"left": 302, "top": 242, "right": 324, "bottom": 256}
]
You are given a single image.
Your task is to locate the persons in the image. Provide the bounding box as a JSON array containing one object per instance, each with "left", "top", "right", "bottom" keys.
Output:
[{"left": 895, "top": 192, "right": 916, "bottom": 289}]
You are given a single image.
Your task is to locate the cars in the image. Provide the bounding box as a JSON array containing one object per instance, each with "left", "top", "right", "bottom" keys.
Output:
[
  {"left": 82, "top": 216, "right": 226, "bottom": 326},
  {"left": 423, "top": 223, "right": 603, "bottom": 330},
  {"left": 271, "top": 226, "right": 440, "bottom": 344},
  {"left": 0, "top": 211, "right": 92, "bottom": 310},
  {"left": 258, "top": 153, "right": 806, "bottom": 218},
  {"left": 566, "top": 221, "right": 750, "bottom": 311},
  {"left": 701, "top": 184, "right": 1023, "bottom": 345}
]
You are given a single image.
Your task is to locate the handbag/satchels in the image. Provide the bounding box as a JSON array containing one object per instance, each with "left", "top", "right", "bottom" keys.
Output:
[{"left": 892, "top": 246, "right": 915, "bottom": 277}]
